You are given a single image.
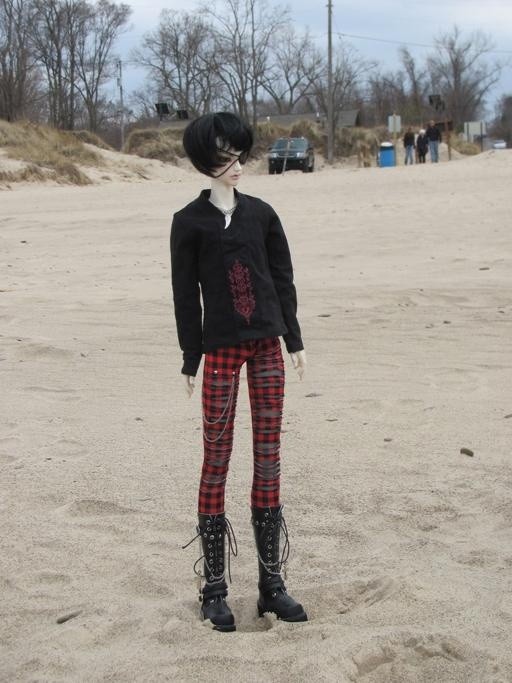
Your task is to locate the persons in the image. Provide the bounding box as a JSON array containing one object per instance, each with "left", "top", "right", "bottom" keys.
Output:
[
  {"left": 404, "top": 120, "right": 442, "bottom": 165},
  {"left": 170, "top": 112, "right": 307, "bottom": 632}
]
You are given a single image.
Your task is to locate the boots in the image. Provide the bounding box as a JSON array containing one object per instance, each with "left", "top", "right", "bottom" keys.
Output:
[
  {"left": 251, "top": 505, "right": 307, "bottom": 621},
  {"left": 182, "top": 512, "right": 237, "bottom": 632}
]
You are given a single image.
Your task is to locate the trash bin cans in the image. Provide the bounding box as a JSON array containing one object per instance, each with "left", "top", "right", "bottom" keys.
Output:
[{"left": 380, "top": 146, "right": 395, "bottom": 167}]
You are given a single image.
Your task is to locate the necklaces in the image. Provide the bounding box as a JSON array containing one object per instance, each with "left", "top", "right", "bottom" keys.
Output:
[{"left": 217, "top": 205, "right": 237, "bottom": 214}]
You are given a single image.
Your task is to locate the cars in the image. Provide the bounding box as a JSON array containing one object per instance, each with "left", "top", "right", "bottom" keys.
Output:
[{"left": 268, "top": 136, "right": 315, "bottom": 174}]
